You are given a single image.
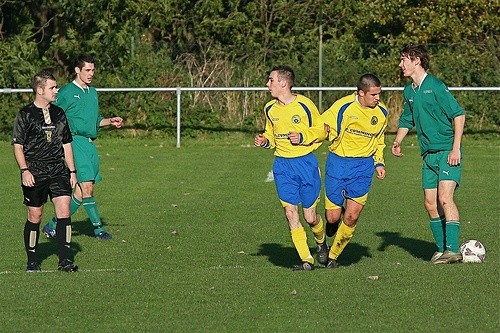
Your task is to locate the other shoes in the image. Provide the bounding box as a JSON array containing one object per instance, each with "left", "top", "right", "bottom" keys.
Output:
[
  {"left": 58, "top": 259, "right": 78, "bottom": 272},
  {"left": 293, "top": 262, "right": 314, "bottom": 271},
  {"left": 325, "top": 219, "right": 341, "bottom": 237},
  {"left": 328, "top": 259, "right": 337, "bottom": 270},
  {"left": 42, "top": 224, "right": 57, "bottom": 240},
  {"left": 315, "top": 242, "right": 328, "bottom": 263},
  {"left": 95, "top": 231, "right": 113, "bottom": 240},
  {"left": 26, "top": 262, "right": 41, "bottom": 273}
]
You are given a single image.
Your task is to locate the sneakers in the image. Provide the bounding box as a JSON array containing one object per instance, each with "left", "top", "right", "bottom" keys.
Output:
[{"left": 430, "top": 251, "right": 463, "bottom": 264}]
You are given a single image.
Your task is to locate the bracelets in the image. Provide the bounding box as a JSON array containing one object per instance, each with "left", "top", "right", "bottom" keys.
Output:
[
  {"left": 21, "top": 168, "right": 28, "bottom": 172},
  {"left": 70, "top": 171, "right": 77, "bottom": 173}
]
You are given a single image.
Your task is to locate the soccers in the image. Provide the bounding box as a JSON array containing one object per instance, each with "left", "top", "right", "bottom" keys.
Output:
[{"left": 459, "top": 239, "right": 487, "bottom": 263}]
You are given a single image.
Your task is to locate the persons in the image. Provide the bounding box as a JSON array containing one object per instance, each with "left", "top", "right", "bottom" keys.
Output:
[
  {"left": 320, "top": 74, "right": 389, "bottom": 269},
  {"left": 42, "top": 54, "right": 123, "bottom": 239},
  {"left": 11, "top": 71, "right": 78, "bottom": 273},
  {"left": 254, "top": 65, "right": 330, "bottom": 270},
  {"left": 392, "top": 43, "right": 465, "bottom": 264}
]
